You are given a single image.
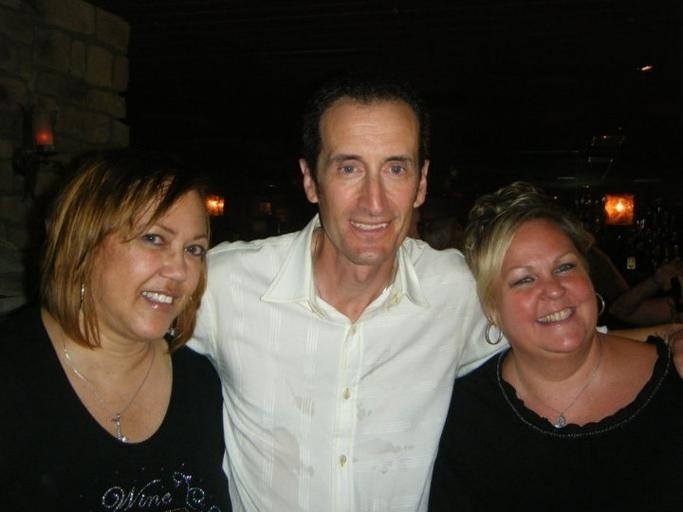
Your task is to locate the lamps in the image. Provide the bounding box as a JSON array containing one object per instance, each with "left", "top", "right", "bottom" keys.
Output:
[{"left": 13, "top": 107, "right": 61, "bottom": 200}]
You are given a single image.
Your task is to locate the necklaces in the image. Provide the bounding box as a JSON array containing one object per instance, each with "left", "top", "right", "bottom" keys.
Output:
[
  {"left": 513, "top": 335, "right": 603, "bottom": 427},
  {"left": 56, "top": 323, "right": 156, "bottom": 442}
]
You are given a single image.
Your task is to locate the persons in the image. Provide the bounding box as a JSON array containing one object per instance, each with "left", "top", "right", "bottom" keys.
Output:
[
  {"left": 171, "top": 76, "right": 683, "bottom": 510},
  {"left": 1, "top": 146, "right": 231, "bottom": 511},
  {"left": 426, "top": 181, "right": 683, "bottom": 512},
  {"left": 608, "top": 252, "right": 682, "bottom": 326}
]
